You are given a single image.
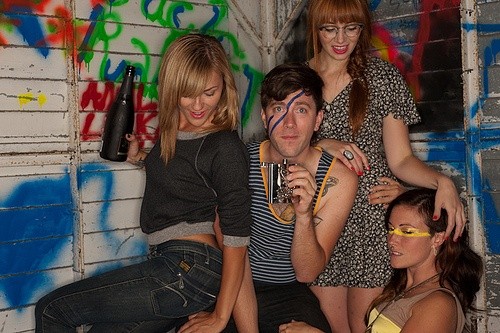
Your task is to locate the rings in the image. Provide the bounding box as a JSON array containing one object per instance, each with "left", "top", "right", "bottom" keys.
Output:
[
  {"left": 286, "top": 323, "right": 288, "bottom": 328},
  {"left": 343, "top": 150, "right": 354, "bottom": 160}
]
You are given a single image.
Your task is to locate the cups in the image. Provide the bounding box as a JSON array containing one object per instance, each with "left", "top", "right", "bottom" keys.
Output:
[{"left": 260, "top": 158, "right": 298, "bottom": 204}]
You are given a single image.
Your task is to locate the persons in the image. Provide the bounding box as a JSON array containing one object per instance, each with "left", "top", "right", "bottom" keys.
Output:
[
  {"left": 278, "top": 177, "right": 483, "bottom": 333},
  {"left": 301, "top": 0, "right": 467, "bottom": 333},
  {"left": 176, "top": 62, "right": 359, "bottom": 333},
  {"left": 35, "top": 31, "right": 254, "bottom": 333}
]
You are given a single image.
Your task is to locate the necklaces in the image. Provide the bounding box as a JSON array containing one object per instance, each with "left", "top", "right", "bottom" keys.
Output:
[{"left": 364, "top": 272, "right": 440, "bottom": 333}]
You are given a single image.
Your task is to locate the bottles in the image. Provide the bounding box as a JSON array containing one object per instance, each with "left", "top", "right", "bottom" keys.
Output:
[{"left": 99, "top": 64, "right": 136, "bottom": 162}]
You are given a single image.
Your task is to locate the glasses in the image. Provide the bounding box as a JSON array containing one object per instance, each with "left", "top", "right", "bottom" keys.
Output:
[{"left": 317, "top": 23, "right": 364, "bottom": 37}]
